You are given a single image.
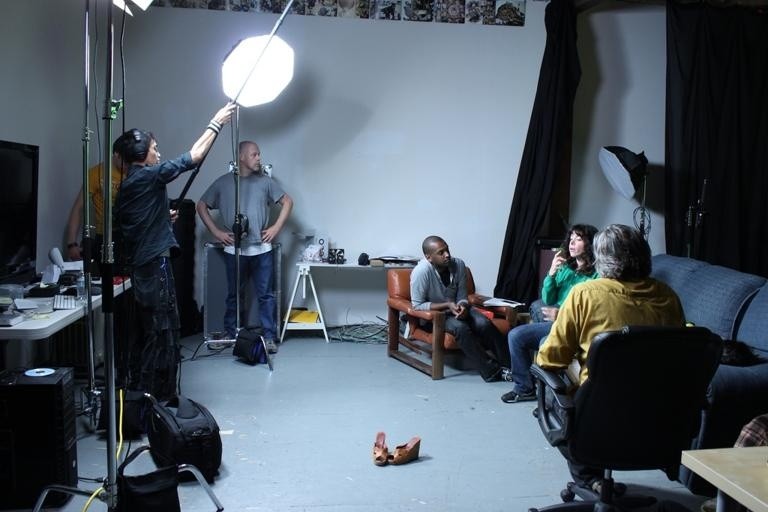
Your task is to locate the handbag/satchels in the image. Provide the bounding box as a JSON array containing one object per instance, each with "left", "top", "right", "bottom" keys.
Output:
[
  {"left": 148, "top": 395, "right": 222, "bottom": 478},
  {"left": 232, "top": 328, "right": 263, "bottom": 366}
]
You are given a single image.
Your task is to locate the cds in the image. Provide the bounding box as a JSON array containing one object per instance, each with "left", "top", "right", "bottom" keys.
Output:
[{"left": 24, "top": 368, "right": 55, "bottom": 377}]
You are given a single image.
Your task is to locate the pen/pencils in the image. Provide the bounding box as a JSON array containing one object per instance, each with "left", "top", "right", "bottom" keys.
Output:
[{"left": 504, "top": 300, "right": 516, "bottom": 304}]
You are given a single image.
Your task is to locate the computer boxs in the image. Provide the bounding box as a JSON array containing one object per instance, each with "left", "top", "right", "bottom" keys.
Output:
[{"left": 0, "top": 366, "right": 78, "bottom": 511}]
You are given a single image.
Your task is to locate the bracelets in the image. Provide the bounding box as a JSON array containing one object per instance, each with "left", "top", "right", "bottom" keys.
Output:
[
  {"left": 68, "top": 243, "right": 78, "bottom": 249},
  {"left": 206, "top": 121, "right": 221, "bottom": 134}
]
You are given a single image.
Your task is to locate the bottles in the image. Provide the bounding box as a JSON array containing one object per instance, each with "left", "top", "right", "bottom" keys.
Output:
[{"left": 76, "top": 272, "right": 88, "bottom": 304}]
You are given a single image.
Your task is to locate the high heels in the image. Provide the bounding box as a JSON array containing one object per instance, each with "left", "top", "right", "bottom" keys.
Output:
[
  {"left": 372, "top": 432, "right": 388, "bottom": 466},
  {"left": 386, "top": 436, "right": 420, "bottom": 464}
]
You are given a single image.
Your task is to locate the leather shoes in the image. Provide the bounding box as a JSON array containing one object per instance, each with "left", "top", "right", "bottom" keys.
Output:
[
  {"left": 592, "top": 480, "right": 627, "bottom": 496},
  {"left": 481, "top": 366, "right": 502, "bottom": 382}
]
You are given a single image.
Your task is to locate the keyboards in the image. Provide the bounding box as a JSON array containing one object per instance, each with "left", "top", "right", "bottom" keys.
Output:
[{"left": 53, "top": 295, "right": 75, "bottom": 310}]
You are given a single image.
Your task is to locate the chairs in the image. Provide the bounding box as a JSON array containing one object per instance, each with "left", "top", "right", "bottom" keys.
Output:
[
  {"left": 528, "top": 324, "right": 722, "bottom": 512},
  {"left": 386, "top": 266, "right": 517, "bottom": 381}
]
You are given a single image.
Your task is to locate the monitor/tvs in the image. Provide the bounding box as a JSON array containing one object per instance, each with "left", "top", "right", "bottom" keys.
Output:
[{"left": 0, "top": 139, "right": 40, "bottom": 284}]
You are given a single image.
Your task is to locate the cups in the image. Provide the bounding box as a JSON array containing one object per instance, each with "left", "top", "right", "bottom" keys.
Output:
[
  {"left": 9, "top": 284, "right": 24, "bottom": 300},
  {"left": 328, "top": 249, "right": 336, "bottom": 264},
  {"left": 336, "top": 248, "right": 344, "bottom": 264}
]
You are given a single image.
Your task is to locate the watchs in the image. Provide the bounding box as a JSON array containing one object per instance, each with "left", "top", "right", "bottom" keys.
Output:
[{"left": 460, "top": 301, "right": 469, "bottom": 307}]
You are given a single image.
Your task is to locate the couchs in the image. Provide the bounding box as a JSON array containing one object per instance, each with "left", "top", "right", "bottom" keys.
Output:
[{"left": 530, "top": 253, "right": 768, "bottom": 495}]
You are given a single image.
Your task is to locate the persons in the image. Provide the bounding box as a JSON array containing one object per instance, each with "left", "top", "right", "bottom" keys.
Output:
[
  {"left": 501, "top": 224, "right": 600, "bottom": 404},
  {"left": 115, "top": 102, "right": 236, "bottom": 399},
  {"left": 533, "top": 224, "right": 685, "bottom": 497},
  {"left": 409, "top": 236, "right": 509, "bottom": 380},
  {"left": 197, "top": 140, "right": 294, "bottom": 354},
  {"left": 66, "top": 137, "right": 125, "bottom": 273}
]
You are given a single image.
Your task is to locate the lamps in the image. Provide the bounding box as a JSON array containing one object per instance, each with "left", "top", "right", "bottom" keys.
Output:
[
  {"left": 174, "top": 34, "right": 295, "bottom": 370},
  {"left": 599, "top": 147, "right": 650, "bottom": 242}
]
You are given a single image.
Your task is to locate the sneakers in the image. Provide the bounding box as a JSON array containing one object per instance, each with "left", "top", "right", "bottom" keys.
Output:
[
  {"left": 265, "top": 339, "right": 276, "bottom": 353},
  {"left": 533, "top": 408, "right": 539, "bottom": 417},
  {"left": 207, "top": 336, "right": 232, "bottom": 350},
  {"left": 502, "top": 391, "right": 537, "bottom": 403}
]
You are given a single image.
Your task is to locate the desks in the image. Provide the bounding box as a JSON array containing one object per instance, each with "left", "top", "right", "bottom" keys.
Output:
[
  {"left": 281, "top": 256, "right": 418, "bottom": 344},
  {"left": 0, "top": 275, "right": 133, "bottom": 370},
  {"left": 680, "top": 447, "right": 768, "bottom": 512}
]
